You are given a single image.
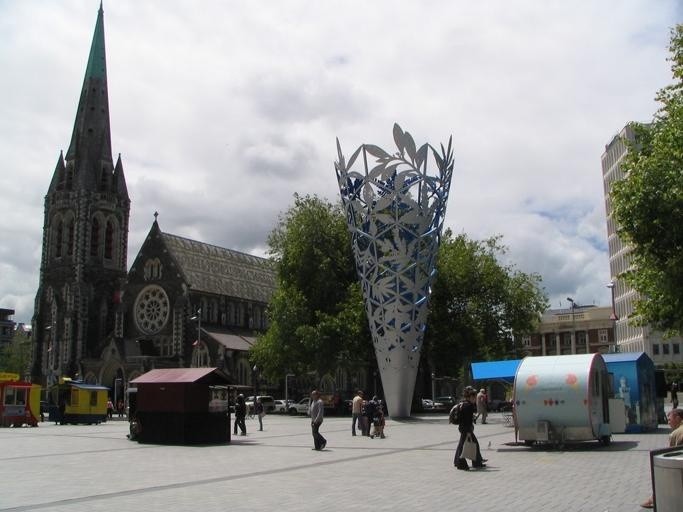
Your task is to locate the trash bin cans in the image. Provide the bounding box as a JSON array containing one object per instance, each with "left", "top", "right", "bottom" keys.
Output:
[{"left": 652, "top": 450, "right": 683, "bottom": 512}]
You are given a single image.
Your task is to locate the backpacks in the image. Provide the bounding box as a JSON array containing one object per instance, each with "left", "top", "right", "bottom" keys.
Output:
[{"left": 449, "top": 403, "right": 461, "bottom": 425}]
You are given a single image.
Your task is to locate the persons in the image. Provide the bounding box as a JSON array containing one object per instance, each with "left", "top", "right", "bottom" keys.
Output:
[
  {"left": 618, "top": 376, "right": 631, "bottom": 425},
  {"left": 670, "top": 382, "right": 679, "bottom": 409},
  {"left": 57, "top": 398, "right": 65, "bottom": 425},
  {"left": 107, "top": 399, "right": 114, "bottom": 419},
  {"left": 454, "top": 386, "right": 489, "bottom": 471},
  {"left": 351, "top": 391, "right": 386, "bottom": 439},
  {"left": 641, "top": 409, "right": 683, "bottom": 508},
  {"left": 116, "top": 399, "right": 124, "bottom": 418},
  {"left": 332, "top": 391, "right": 343, "bottom": 416},
  {"left": 309, "top": 390, "right": 327, "bottom": 450},
  {"left": 233, "top": 393, "right": 264, "bottom": 436}
]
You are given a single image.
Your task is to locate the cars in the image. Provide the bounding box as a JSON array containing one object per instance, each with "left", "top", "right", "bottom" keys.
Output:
[
  {"left": 421, "top": 398, "right": 448, "bottom": 412},
  {"left": 436, "top": 396, "right": 457, "bottom": 413},
  {"left": 245, "top": 391, "right": 353, "bottom": 416}
]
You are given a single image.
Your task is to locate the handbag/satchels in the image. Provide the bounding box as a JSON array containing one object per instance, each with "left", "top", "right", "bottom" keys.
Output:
[{"left": 459, "top": 435, "right": 478, "bottom": 461}]
